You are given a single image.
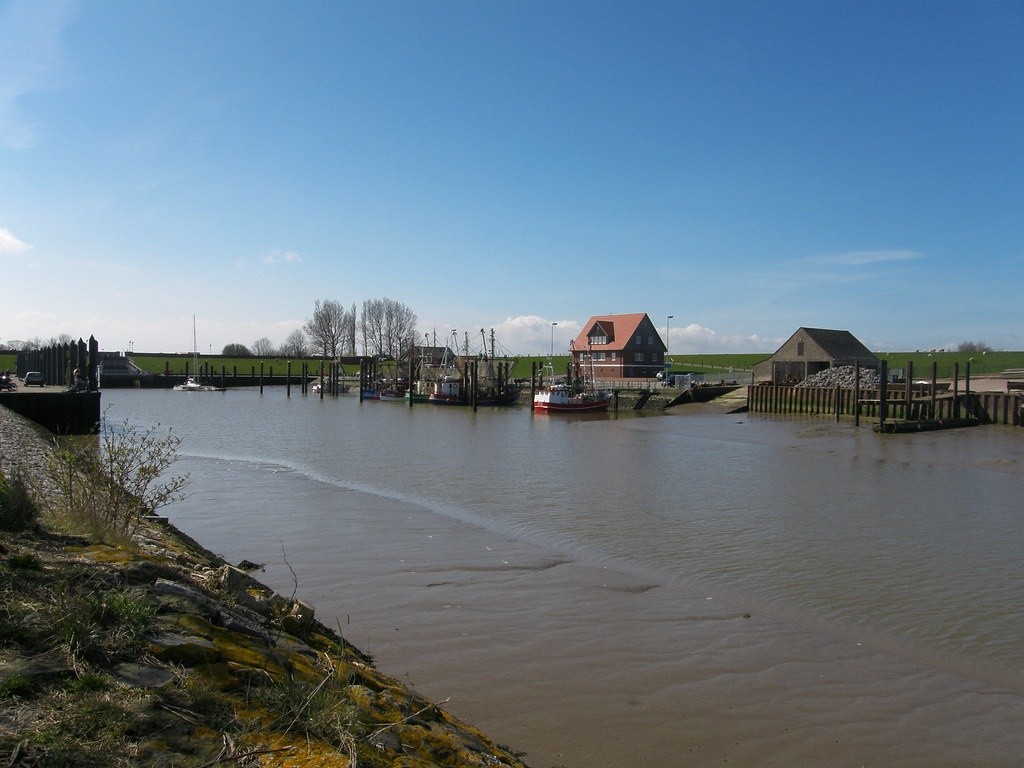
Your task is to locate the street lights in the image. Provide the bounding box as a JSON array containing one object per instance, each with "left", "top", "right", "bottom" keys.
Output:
[
  {"left": 551, "top": 322, "right": 557, "bottom": 366},
  {"left": 666, "top": 315, "right": 673, "bottom": 389},
  {"left": 132, "top": 342, "right": 134, "bottom": 353},
  {"left": 209, "top": 344, "right": 212, "bottom": 356},
  {"left": 450, "top": 329, "right": 456, "bottom": 367}
]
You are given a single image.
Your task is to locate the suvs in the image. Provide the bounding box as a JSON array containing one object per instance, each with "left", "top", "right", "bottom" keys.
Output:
[{"left": 656, "top": 372, "right": 671, "bottom": 380}]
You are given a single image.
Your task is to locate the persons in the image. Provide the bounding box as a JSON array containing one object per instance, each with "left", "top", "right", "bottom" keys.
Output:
[
  {"left": 3, "top": 369, "right": 16, "bottom": 392},
  {"left": 69, "top": 368, "right": 82, "bottom": 392},
  {"left": 24, "top": 367, "right": 30, "bottom": 377}
]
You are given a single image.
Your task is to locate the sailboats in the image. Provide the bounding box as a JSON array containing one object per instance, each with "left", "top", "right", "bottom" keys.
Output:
[
  {"left": 363, "top": 328, "right": 527, "bottom": 405},
  {"left": 173, "top": 314, "right": 227, "bottom": 391}
]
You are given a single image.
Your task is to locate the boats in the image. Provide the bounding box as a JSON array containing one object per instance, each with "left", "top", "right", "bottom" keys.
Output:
[
  {"left": 533, "top": 354, "right": 615, "bottom": 413},
  {"left": 312, "top": 380, "right": 353, "bottom": 393}
]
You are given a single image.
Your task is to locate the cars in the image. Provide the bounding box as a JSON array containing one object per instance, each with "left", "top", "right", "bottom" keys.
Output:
[
  {"left": 24, "top": 372, "right": 44, "bottom": 387},
  {"left": 661, "top": 375, "right": 687, "bottom": 388}
]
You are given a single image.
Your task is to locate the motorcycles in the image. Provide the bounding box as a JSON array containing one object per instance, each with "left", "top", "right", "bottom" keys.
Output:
[{"left": 0, "top": 370, "right": 18, "bottom": 391}]
